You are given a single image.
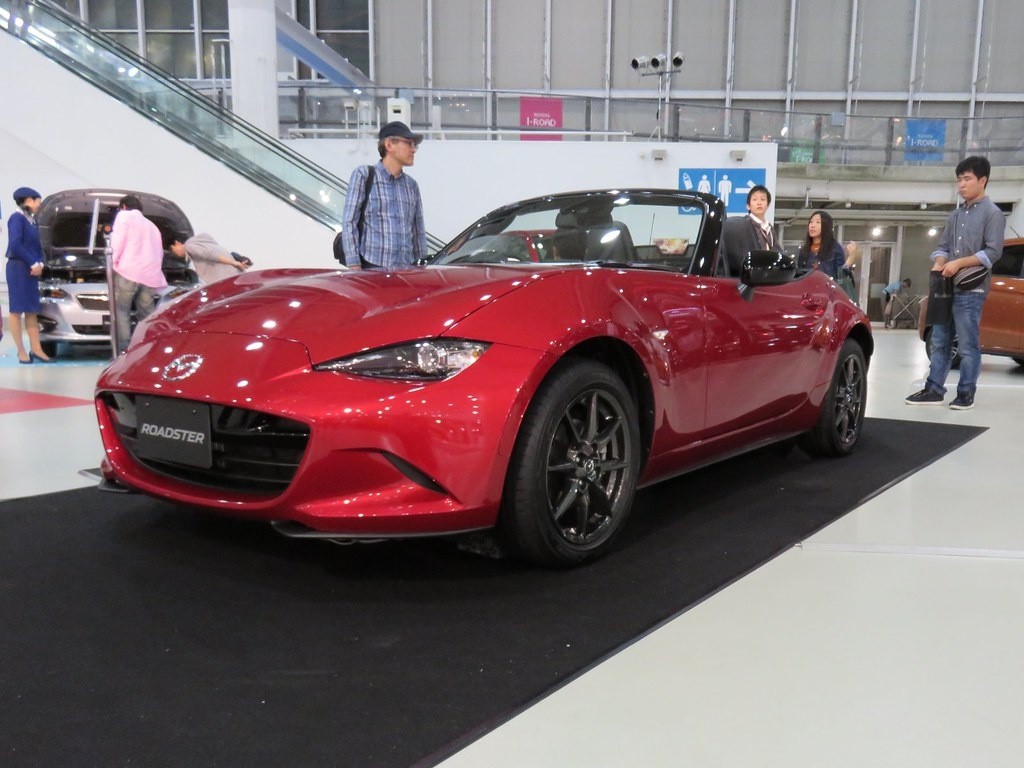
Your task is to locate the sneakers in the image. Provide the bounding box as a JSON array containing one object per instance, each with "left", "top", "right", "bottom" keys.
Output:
[
  {"left": 949, "top": 386, "right": 977, "bottom": 409},
  {"left": 905, "top": 389, "right": 944, "bottom": 406}
]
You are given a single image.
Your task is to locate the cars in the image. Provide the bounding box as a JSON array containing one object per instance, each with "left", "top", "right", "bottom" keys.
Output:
[
  {"left": 917, "top": 236, "right": 1024, "bottom": 370},
  {"left": 27, "top": 191, "right": 200, "bottom": 356}
]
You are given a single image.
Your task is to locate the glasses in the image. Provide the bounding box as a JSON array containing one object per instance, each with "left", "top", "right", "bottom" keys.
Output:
[{"left": 398, "top": 139, "right": 419, "bottom": 149}]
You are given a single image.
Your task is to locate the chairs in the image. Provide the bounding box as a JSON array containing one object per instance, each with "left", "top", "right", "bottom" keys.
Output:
[{"left": 585, "top": 221, "right": 638, "bottom": 263}]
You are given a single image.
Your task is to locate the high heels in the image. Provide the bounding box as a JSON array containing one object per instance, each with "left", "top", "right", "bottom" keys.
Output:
[
  {"left": 16, "top": 353, "right": 32, "bottom": 363},
  {"left": 29, "top": 351, "right": 56, "bottom": 363}
]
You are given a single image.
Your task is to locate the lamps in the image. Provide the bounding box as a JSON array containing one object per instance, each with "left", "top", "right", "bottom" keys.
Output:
[
  {"left": 631, "top": 56, "right": 650, "bottom": 70},
  {"left": 672, "top": 51, "right": 683, "bottom": 68},
  {"left": 651, "top": 53, "right": 667, "bottom": 68}
]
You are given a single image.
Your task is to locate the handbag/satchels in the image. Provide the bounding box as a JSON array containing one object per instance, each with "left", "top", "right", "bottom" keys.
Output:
[
  {"left": 231, "top": 252, "right": 253, "bottom": 265},
  {"left": 333, "top": 221, "right": 362, "bottom": 267},
  {"left": 925, "top": 270, "right": 953, "bottom": 324},
  {"left": 952, "top": 265, "right": 989, "bottom": 290},
  {"left": 836, "top": 266, "right": 860, "bottom": 307}
]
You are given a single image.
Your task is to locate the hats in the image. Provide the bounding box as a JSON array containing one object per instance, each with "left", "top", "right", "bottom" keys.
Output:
[
  {"left": 13, "top": 187, "right": 41, "bottom": 199},
  {"left": 379, "top": 120, "right": 423, "bottom": 144}
]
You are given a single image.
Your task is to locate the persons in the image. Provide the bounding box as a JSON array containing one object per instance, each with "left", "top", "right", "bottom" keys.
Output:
[
  {"left": 167, "top": 231, "right": 250, "bottom": 284},
  {"left": 4, "top": 187, "right": 56, "bottom": 364},
  {"left": 904, "top": 156, "right": 1006, "bottom": 410},
  {"left": 110, "top": 194, "right": 168, "bottom": 355},
  {"left": 342, "top": 121, "right": 428, "bottom": 270},
  {"left": 880, "top": 278, "right": 911, "bottom": 329},
  {"left": 798, "top": 210, "right": 846, "bottom": 284},
  {"left": 744, "top": 185, "right": 784, "bottom": 252}
]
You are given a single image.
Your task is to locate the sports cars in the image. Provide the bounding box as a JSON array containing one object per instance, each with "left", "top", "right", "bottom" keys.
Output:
[{"left": 93, "top": 186, "right": 875, "bottom": 570}]
